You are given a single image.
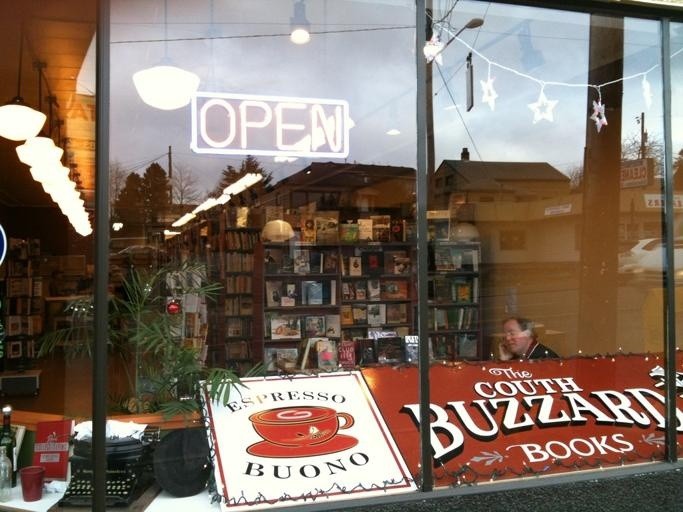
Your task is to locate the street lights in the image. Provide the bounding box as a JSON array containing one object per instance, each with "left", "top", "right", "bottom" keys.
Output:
[{"left": 425, "top": 14, "right": 484, "bottom": 213}]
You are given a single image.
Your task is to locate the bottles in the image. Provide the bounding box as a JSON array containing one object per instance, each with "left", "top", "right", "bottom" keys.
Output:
[{"left": 0, "top": 405, "right": 18, "bottom": 505}]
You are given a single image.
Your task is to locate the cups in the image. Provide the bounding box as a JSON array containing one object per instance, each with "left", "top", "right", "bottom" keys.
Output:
[{"left": 19, "top": 466, "right": 45, "bottom": 503}]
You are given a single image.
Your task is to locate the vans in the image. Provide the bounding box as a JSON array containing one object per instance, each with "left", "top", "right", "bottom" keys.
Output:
[{"left": 110, "top": 236, "right": 149, "bottom": 252}]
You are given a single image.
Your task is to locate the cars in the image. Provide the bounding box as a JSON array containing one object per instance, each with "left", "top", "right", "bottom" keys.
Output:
[
  {"left": 618, "top": 238, "right": 683, "bottom": 272},
  {"left": 110, "top": 246, "right": 170, "bottom": 268},
  {"left": 31, "top": 256, "right": 92, "bottom": 292}
]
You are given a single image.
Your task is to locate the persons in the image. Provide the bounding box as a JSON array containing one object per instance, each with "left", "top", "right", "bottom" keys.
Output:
[{"left": 499, "top": 317, "right": 559, "bottom": 361}]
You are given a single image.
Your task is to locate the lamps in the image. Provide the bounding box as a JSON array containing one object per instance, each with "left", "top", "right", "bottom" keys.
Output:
[
  {"left": 289, "top": 0, "right": 311, "bottom": 45},
  {"left": 133, "top": 0, "right": 201, "bottom": 111},
  {"left": 0, "top": 24, "right": 93, "bottom": 238}
]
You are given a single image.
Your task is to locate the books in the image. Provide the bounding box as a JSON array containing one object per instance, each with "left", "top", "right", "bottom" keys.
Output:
[
  {"left": 224, "top": 230, "right": 259, "bottom": 340},
  {"left": 32, "top": 419, "right": 73, "bottom": 482},
  {"left": 458, "top": 308, "right": 479, "bottom": 330}
]
